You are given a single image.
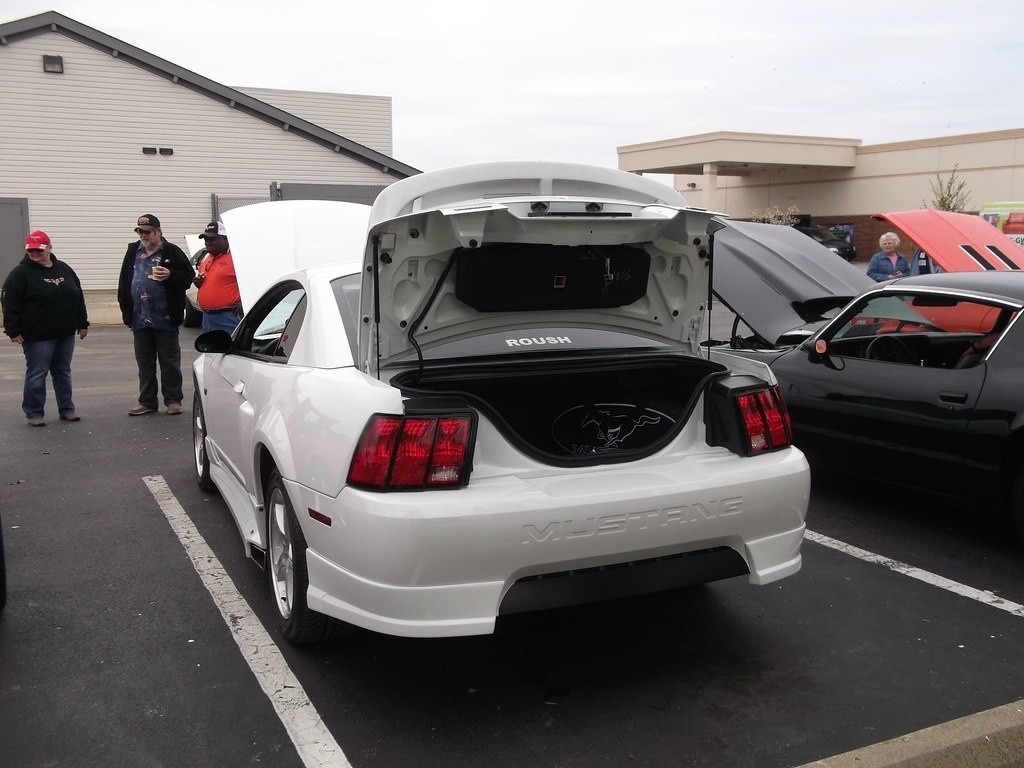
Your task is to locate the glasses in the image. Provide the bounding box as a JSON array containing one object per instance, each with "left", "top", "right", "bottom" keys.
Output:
[
  {"left": 137, "top": 230, "right": 151, "bottom": 235},
  {"left": 27, "top": 246, "right": 48, "bottom": 253}
]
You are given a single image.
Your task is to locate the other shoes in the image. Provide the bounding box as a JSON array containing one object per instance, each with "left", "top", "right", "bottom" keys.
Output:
[
  {"left": 128, "top": 405, "right": 154, "bottom": 415},
  {"left": 29, "top": 416, "right": 46, "bottom": 426},
  {"left": 60, "top": 409, "right": 80, "bottom": 422},
  {"left": 167, "top": 403, "right": 183, "bottom": 414}
]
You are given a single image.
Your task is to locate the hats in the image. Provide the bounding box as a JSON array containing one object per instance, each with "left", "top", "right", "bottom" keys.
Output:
[
  {"left": 135, "top": 214, "right": 160, "bottom": 231},
  {"left": 24, "top": 230, "right": 50, "bottom": 249},
  {"left": 199, "top": 222, "right": 227, "bottom": 239}
]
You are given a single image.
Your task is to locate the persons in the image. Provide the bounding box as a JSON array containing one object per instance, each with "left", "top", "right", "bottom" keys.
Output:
[
  {"left": 1, "top": 231, "right": 90, "bottom": 427},
  {"left": 117, "top": 214, "right": 196, "bottom": 414},
  {"left": 866, "top": 232, "right": 910, "bottom": 283},
  {"left": 193, "top": 222, "right": 244, "bottom": 335}
]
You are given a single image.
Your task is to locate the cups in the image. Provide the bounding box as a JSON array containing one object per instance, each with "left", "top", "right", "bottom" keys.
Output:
[{"left": 152, "top": 267, "right": 163, "bottom": 280}]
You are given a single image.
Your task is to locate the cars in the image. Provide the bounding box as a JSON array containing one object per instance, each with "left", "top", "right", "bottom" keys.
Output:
[
  {"left": 794, "top": 227, "right": 858, "bottom": 264},
  {"left": 699, "top": 219, "right": 1023, "bottom": 562},
  {"left": 845, "top": 204, "right": 1024, "bottom": 352},
  {"left": 191, "top": 159, "right": 811, "bottom": 651},
  {"left": 182, "top": 233, "right": 206, "bottom": 330}
]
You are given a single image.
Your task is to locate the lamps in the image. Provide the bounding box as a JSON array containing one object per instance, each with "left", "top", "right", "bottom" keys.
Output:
[{"left": 42, "top": 55, "right": 63, "bottom": 74}]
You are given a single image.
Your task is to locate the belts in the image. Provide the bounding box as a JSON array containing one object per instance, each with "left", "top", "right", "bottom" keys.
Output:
[{"left": 204, "top": 308, "right": 233, "bottom": 314}]
